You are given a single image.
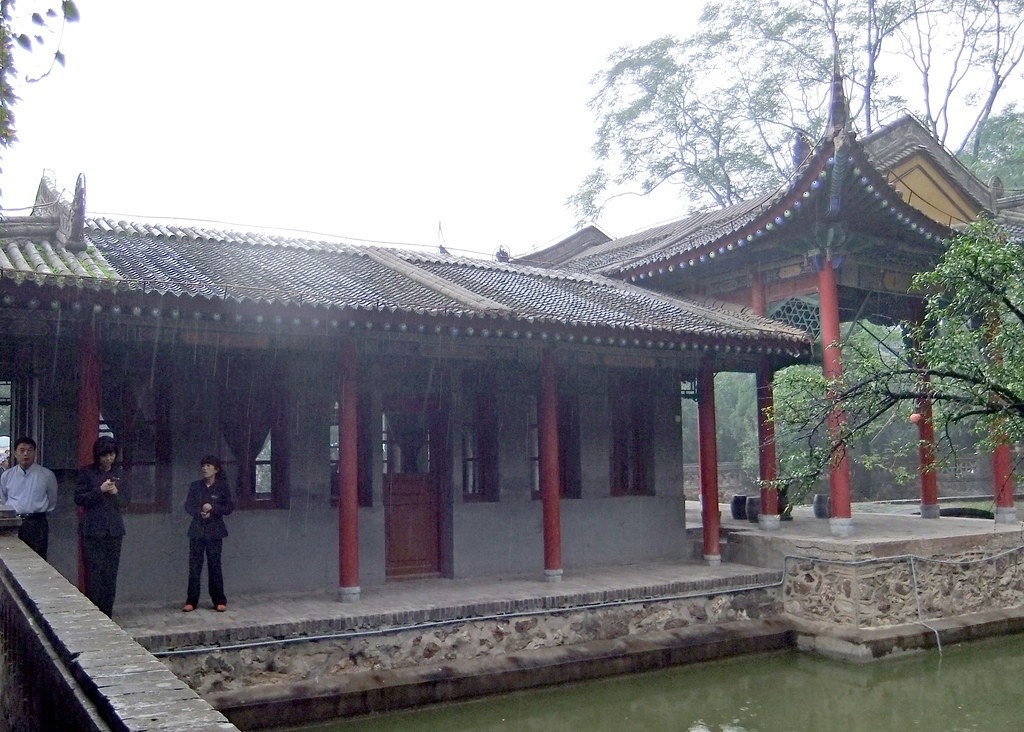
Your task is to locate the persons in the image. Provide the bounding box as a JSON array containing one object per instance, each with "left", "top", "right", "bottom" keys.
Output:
[
  {"left": 74, "top": 436, "right": 131, "bottom": 618},
  {"left": 0, "top": 435, "right": 58, "bottom": 562},
  {"left": 182, "top": 455, "right": 234, "bottom": 612}
]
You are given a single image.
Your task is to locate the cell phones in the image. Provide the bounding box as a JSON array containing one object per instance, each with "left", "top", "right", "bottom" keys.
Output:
[{"left": 110, "top": 478, "right": 120, "bottom": 482}]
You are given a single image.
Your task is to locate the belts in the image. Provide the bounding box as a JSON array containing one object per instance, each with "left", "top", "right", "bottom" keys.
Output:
[{"left": 15, "top": 513, "right": 30, "bottom": 521}]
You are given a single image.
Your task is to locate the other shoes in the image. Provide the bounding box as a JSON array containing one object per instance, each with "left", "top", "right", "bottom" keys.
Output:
[
  {"left": 216, "top": 605, "right": 226, "bottom": 612},
  {"left": 182, "top": 604, "right": 193, "bottom": 612}
]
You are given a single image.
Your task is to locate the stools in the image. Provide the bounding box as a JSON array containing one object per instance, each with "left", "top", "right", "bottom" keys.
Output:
[
  {"left": 746, "top": 496, "right": 760, "bottom": 523},
  {"left": 813, "top": 494, "right": 830, "bottom": 518},
  {"left": 731, "top": 495, "right": 748, "bottom": 520}
]
